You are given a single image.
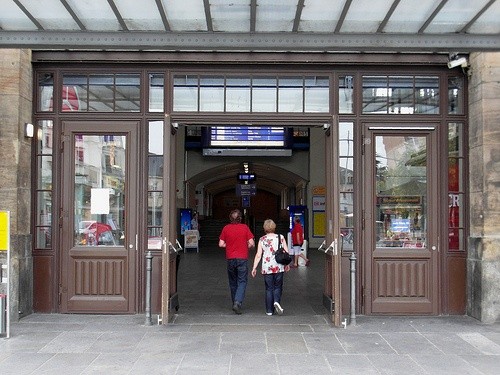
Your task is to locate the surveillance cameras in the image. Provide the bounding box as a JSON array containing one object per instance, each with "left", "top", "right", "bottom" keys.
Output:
[{"left": 448, "top": 56, "right": 467, "bottom": 69}]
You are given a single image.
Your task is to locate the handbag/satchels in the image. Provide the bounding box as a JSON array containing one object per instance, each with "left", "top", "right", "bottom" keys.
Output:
[{"left": 275, "top": 234, "right": 292, "bottom": 265}]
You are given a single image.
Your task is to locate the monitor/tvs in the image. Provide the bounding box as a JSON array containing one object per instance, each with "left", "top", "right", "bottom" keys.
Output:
[{"left": 206, "top": 126, "right": 293, "bottom": 149}]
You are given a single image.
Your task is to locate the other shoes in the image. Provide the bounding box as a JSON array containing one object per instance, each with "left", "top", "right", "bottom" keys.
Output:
[
  {"left": 305, "top": 260, "right": 310, "bottom": 266},
  {"left": 273, "top": 302, "right": 283, "bottom": 314},
  {"left": 290, "top": 264, "right": 297, "bottom": 268},
  {"left": 265, "top": 312, "right": 273, "bottom": 316},
  {"left": 231, "top": 304, "right": 242, "bottom": 315}
]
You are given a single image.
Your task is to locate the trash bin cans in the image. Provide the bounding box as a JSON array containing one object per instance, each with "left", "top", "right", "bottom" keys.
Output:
[{"left": 0, "top": 294, "right": 6, "bottom": 335}]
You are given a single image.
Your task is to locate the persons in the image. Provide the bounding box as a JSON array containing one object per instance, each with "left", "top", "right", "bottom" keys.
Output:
[
  {"left": 191, "top": 213, "right": 199, "bottom": 252},
  {"left": 290, "top": 216, "right": 310, "bottom": 267},
  {"left": 181, "top": 221, "right": 190, "bottom": 231},
  {"left": 219, "top": 210, "right": 255, "bottom": 314},
  {"left": 251, "top": 219, "right": 291, "bottom": 315}
]
